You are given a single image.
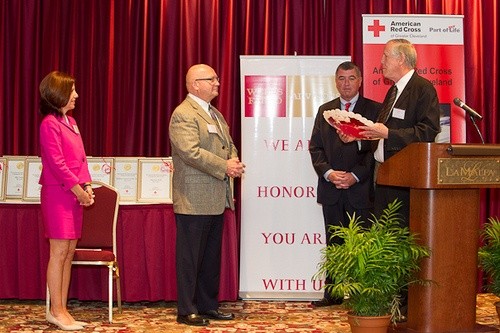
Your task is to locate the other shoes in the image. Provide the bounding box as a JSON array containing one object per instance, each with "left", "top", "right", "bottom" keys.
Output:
[{"left": 311, "top": 298, "right": 333, "bottom": 307}]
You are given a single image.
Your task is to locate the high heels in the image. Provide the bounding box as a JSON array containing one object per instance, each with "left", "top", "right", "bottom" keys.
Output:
[{"left": 46, "top": 313, "right": 84, "bottom": 330}]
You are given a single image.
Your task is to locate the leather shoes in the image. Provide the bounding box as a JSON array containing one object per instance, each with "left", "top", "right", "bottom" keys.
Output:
[
  {"left": 205, "top": 310, "right": 235, "bottom": 319},
  {"left": 177, "top": 313, "right": 210, "bottom": 325}
]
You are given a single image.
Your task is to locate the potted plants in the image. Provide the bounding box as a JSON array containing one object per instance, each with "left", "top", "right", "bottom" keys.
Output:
[
  {"left": 310, "top": 196, "right": 432, "bottom": 333},
  {"left": 479, "top": 214, "right": 500, "bottom": 321}
]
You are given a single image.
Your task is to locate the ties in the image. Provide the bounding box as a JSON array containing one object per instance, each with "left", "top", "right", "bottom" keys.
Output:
[
  {"left": 345, "top": 102, "right": 351, "bottom": 112},
  {"left": 209, "top": 104, "right": 222, "bottom": 133},
  {"left": 372, "top": 85, "right": 398, "bottom": 153}
]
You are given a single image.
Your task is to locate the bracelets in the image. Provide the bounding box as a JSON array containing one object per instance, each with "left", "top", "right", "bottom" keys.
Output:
[{"left": 84, "top": 183, "right": 91, "bottom": 187}]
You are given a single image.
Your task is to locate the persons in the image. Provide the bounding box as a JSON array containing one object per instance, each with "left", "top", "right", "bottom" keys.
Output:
[
  {"left": 310, "top": 62, "right": 383, "bottom": 306},
  {"left": 30, "top": 71, "right": 95, "bottom": 330},
  {"left": 338, "top": 39, "right": 442, "bottom": 321},
  {"left": 169, "top": 63, "right": 246, "bottom": 325}
]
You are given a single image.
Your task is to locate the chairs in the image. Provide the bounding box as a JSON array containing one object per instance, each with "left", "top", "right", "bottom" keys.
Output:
[{"left": 45, "top": 181, "right": 121, "bottom": 323}]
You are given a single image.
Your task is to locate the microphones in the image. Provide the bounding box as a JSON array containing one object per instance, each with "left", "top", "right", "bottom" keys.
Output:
[{"left": 454, "top": 97, "right": 483, "bottom": 120}]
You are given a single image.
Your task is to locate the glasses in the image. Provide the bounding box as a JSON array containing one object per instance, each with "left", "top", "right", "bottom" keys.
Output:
[{"left": 196, "top": 77, "right": 219, "bottom": 82}]
